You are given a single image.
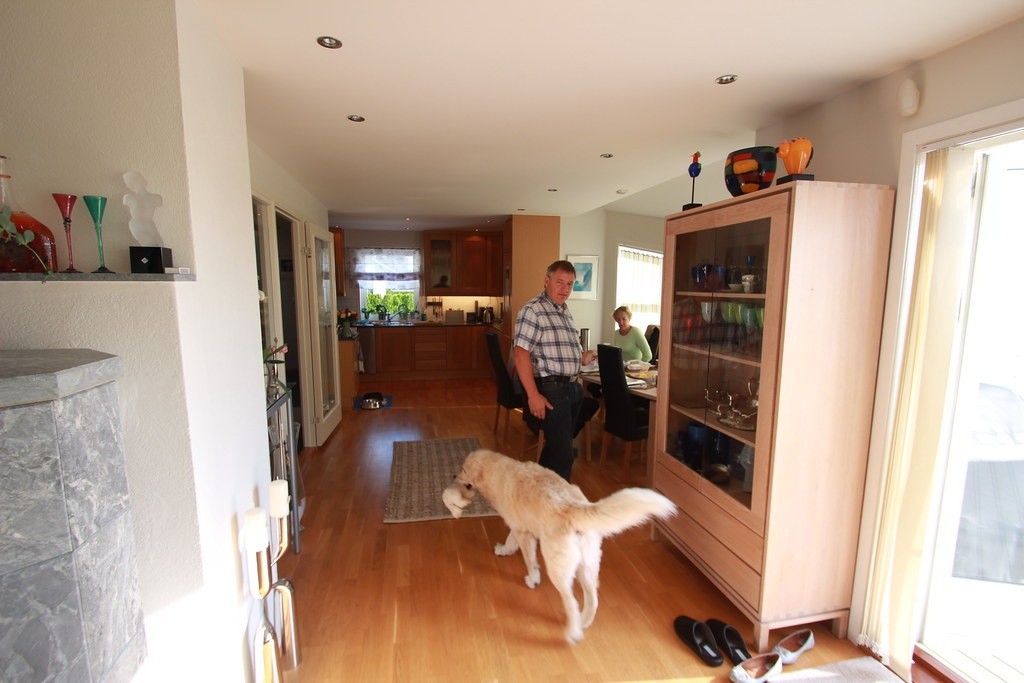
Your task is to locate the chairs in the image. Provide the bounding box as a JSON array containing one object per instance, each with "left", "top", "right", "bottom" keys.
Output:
[
  {"left": 486, "top": 332, "right": 592, "bottom": 463},
  {"left": 644, "top": 325, "right": 660, "bottom": 365},
  {"left": 597, "top": 344, "right": 650, "bottom": 477}
]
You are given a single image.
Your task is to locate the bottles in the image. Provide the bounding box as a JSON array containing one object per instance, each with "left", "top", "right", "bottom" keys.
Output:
[
  {"left": 693, "top": 255, "right": 761, "bottom": 294},
  {"left": 0, "top": 154, "right": 58, "bottom": 274}
]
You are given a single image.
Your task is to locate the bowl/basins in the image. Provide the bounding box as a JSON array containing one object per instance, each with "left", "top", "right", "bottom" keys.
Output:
[{"left": 362, "top": 398, "right": 381, "bottom": 410}]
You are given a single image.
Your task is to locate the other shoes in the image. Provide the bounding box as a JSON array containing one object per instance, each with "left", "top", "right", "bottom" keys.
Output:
[
  {"left": 727, "top": 652, "right": 782, "bottom": 683},
  {"left": 771, "top": 628, "right": 815, "bottom": 664}
]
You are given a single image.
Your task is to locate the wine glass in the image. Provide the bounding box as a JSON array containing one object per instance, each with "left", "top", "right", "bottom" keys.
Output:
[
  {"left": 50, "top": 192, "right": 85, "bottom": 273},
  {"left": 677, "top": 300, "right": 767, "bottom": 358},
  {"left": 83, "top": 195, "right": 117, "bottom": 274}
]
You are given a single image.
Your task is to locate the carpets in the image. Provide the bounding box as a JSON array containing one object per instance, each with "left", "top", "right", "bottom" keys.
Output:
[
  {"left": 382, "top": 437, "right": 499, "bottom": 523},
  {"left": 768, "top": 656, "right": 906, "bottom": 683},
  {"left": 353, "top": 396, "right": 393, "bottom": 409}
]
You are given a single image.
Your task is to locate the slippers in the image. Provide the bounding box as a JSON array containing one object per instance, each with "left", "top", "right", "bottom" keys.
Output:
[
  {"left": 708, "top": 618, "right": 752, "bottom": 666},
  {"left": 675, "top": 614, "right": 723, "bottom": 666}
]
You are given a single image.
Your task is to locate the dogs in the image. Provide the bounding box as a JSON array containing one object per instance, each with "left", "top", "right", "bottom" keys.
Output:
[{"left": 454, "top": 449, "right": 680, "bottom": 644}]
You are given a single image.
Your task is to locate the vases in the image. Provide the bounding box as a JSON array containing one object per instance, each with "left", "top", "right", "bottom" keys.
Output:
[
  {"left": 725, "top": 146, "right": 777, "bottom": 197},
  {"left": 341, "top": 321, "right": 353, "bottom": 338},
  {"left": 264, "top": 362, "right": 273, "bottom": 391}
]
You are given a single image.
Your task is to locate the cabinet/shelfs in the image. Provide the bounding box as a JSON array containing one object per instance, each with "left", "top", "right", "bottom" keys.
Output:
[
  {"left": 421, "top": 230, "right": 486, "bottom": 296},
  {"left": 503, "top": 215, "right": 560, "bottom": 340},
  {"left": 486, "top": 231, "right": 503, "bottom": 297},
  {"left": 329, "top": 226, "right": 347, "bottom": 297},
  {"left": 647, "top": 180, "right": 897, "bottom": 653},
  {"left": 357, "top": 326, "right": 516, "bottom": 382},
  {"left": 339, "top": 337, "right": 361, "bottom": 411}
]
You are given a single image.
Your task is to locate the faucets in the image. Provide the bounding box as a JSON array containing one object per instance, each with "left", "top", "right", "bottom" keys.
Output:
[{"left": 386, "top": 310, "right": 403, "bottom": 322}]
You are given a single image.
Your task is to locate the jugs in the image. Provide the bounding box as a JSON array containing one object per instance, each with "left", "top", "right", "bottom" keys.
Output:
[{"left": 483, "top": 309, "right": 493, "bottom": 323}]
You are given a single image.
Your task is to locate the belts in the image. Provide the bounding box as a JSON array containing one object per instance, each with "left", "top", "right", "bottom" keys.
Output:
[{"left": 536, "top": 375, "right": 578, "bottom": 382}]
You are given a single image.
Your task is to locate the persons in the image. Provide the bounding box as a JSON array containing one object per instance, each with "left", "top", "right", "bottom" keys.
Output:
[
  {"left": 433, "top": 275, "right": 450, "bottom": 288},
  {"left": 122, "top": 172, "right": 165, "bottom": 247},
  {"left": 612, "top": 306, "right": 652, "bottom": 362},
  {"left": 514, "top": 260, "right": 598, "bottom": 484}
]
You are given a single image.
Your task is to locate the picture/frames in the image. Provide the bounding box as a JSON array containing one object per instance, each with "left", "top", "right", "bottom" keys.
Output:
[{"left": 566, "top": 255, "right": 599, "bottom": 301}]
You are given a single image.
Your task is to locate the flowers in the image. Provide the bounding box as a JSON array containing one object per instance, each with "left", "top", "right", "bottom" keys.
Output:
[
  {"left": 337, "top": 307, "right": 358, "bottom": 324},
  {"left": 262, "top": 337, "right": 291, "bottom": 399}
]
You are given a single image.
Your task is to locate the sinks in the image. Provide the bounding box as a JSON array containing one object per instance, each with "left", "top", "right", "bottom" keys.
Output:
[{"left": 365, "top": 321, "right": 415, "bottom": 326}]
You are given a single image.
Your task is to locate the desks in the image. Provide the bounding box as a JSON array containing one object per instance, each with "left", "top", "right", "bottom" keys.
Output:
[
  {"left": 578, "top": 373, "right": 657, "bottom": 491},
  {"left": 266, "top": 382, "right": 300, "bottom": 554}
]
